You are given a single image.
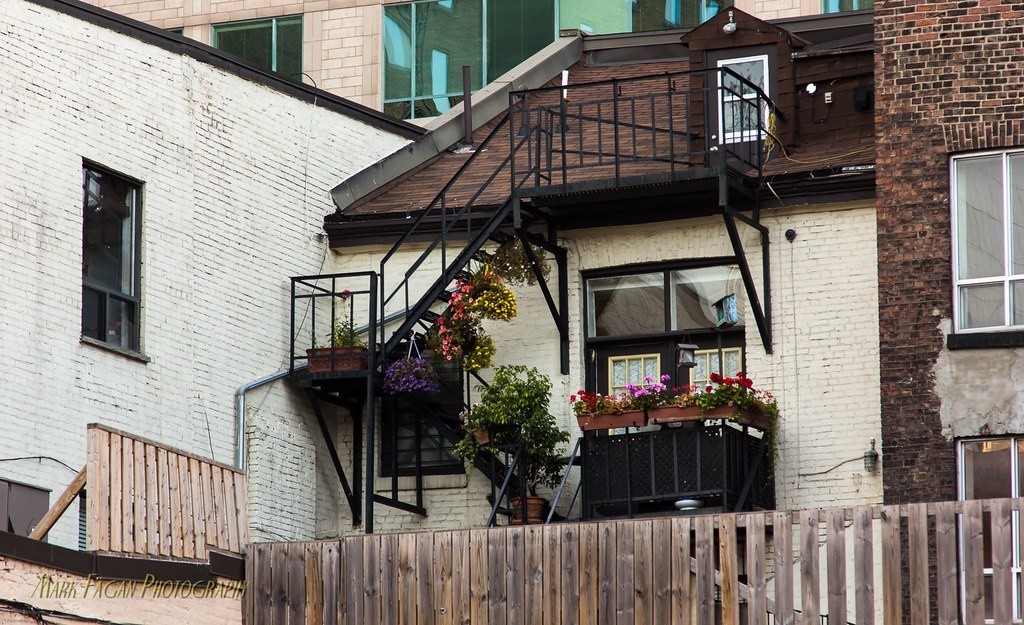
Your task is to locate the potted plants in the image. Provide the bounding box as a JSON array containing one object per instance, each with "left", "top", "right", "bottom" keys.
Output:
[
  {"left": 448, "top": 363, "right": 571, "bottom": 523},
  {"left": 305, "top": 289, "right": 371, "bottom": 373},
  {"left": 568, "top": 373, "right": 779, "bottom": 487}
]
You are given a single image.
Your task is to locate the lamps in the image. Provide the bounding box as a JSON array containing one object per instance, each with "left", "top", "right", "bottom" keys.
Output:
[{"left": 865, "top": 441, "right": 879, "bottom": 475}]
